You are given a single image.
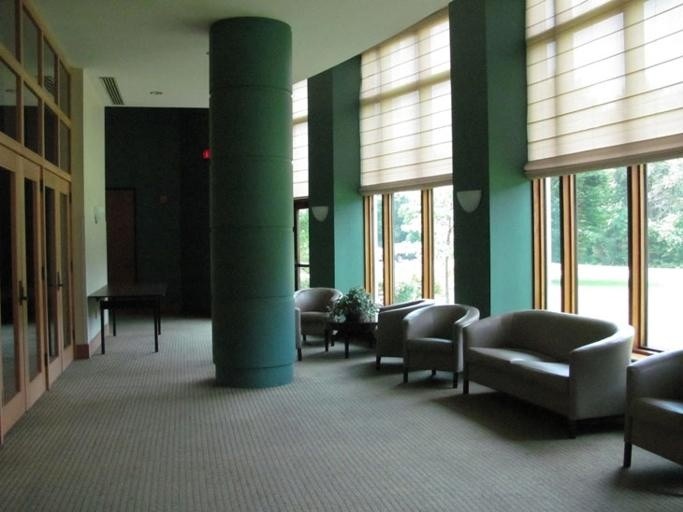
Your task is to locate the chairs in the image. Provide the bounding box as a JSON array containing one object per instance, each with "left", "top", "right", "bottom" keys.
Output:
[
  {"left": 376, "top": 299, "right": 480, "bottom": 388},
  {"left": 293, "top": 287, "right": 341, "bottom": 351},
  {"left": 625, "top": 349, "right": 683, "bottom": 467}
]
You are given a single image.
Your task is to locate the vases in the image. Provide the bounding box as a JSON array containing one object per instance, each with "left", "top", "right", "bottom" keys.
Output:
[{"left": 326, "top": 286, "right": 381, "bottom": 322}]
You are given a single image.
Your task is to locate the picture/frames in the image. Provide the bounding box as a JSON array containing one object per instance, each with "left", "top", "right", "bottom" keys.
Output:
[{"left": 106, "top": 187, "right": 137, "bottom": 284}]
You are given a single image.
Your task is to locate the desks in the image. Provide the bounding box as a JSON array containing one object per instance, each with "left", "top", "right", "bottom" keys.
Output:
[{"left": 88, "top": 272, "right": 170, "bottom": 354}]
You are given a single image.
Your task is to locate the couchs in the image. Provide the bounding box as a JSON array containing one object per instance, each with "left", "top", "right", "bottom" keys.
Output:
[{"left": 463, "top": 309, "right": 636, "bottom": 439}]
